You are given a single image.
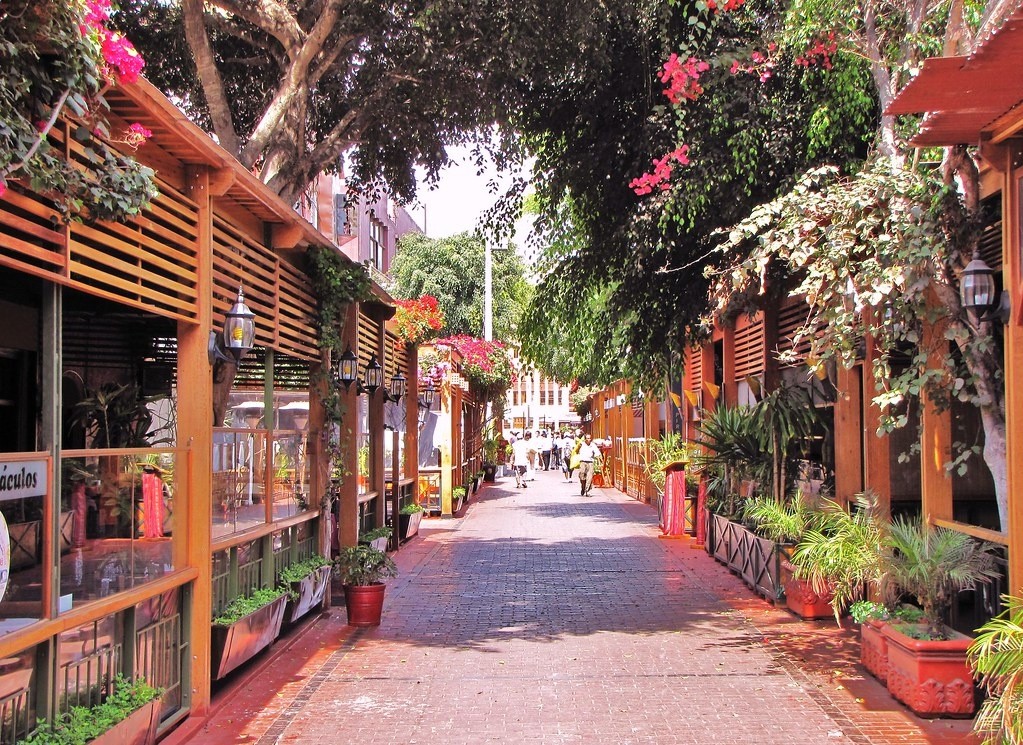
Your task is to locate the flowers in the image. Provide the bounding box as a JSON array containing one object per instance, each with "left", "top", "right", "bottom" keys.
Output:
[
  {"left": 391, "top": 294, "right": 444, "bottom": 347},
  {"left": 418, "top": 334, "right": 517, "bottom": 401},
  {"left": 0, "top": 0, "right": 158, "bottom": 223}
]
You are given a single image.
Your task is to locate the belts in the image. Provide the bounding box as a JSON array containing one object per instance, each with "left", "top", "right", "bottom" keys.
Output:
[{"left": 580, "top": 460, "right": 593, "bottom": 463}]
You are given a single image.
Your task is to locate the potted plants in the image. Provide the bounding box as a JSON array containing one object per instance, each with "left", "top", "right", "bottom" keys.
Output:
[
  {"left": 211, "top": 586, "right": 300, "bottom": 680},
  {"left": 462, "top": 439, "right": 500, "bottom": 501},
  {"left": 277, "top": 552, "right": 335, "bottom": 623},
  {"left": 61, "top": 380, "right": 175, "bottom": 537},
  {"left": 399, "top": 503, "right": 425, "bottom": 538},
  {"left": 452, "top": 485, "right": 466, "bottom": 512},
  {"left": 17, "top": 672, "right": 166, "bottom": 745},
  {"left": 358, "top": 528, "right": 394, "bottom": 552},
  {"left": 626, "top": 381, "right": 1007, "bottom": 719},
  {"left": 336, "top": 545, "right": 399, "bottom": 627}
]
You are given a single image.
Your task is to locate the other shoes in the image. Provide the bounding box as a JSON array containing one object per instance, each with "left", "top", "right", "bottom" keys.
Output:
[
  {"left": 581, "top": 490, "right": 585, "bottom": 496},
  {"left": 586, "top": 490, "right": 590, "bottom": 497},
  {"left": 522, "top": 484, "right": 527, "bottom": 488},
  {"left": 569, "top": 480, "right": 572, "bottom": 483},
  {"left": 546, "top": 469, "right": 549, "bottom": 471},
  {"left": 543, "top": 469, "right": 546, "bottom": 471},
  {"left": 516, "top": 486, "right": 519, "bottom": 488}
]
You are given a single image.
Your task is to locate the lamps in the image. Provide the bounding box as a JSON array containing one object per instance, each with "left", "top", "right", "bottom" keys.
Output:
[
  {"left": 357, "top": 351, "right": 382, "bottom": 397},
  {"left": 583, "top": 408, "right": 600, "bottom": 424},
  {"left": 330, "top": 338, "right": 358, "bottom": 394},
  {"left": 959, "top": 242, "right": 1011, "bottom": 329},
  {"left": 418, "top": 383, "right": 436, "bottom": 409},
  {"left": 208, "top": 285, "right": 256, "bottom": 370},
  {"left": 884, "top": 290, "right": 923, "bottom": 352},
  {"left": 384, "top": 365, "right": 406, "bottom": 406}
]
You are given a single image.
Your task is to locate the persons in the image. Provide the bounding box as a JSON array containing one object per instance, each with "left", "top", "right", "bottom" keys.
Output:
[
  {"left": 576, "top": 434, "right": 600, "bottom": 497},
  {"left": 508, "top": 430, "right": 584, "bottom": 488}
]
